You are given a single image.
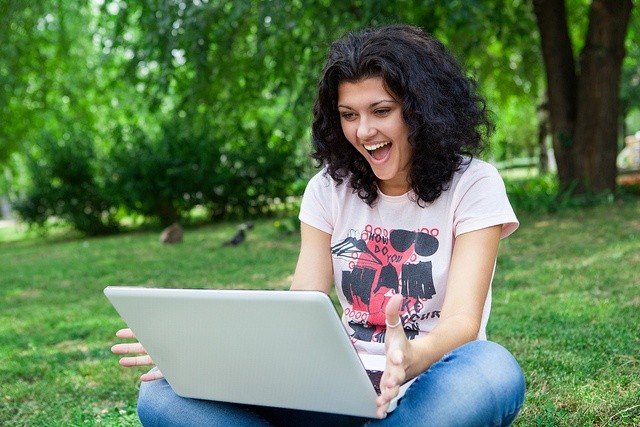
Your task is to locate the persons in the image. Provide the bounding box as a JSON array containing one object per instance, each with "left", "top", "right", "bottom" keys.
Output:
[{"left": 110, "top": 23, "right": 526, "bottom": 427}]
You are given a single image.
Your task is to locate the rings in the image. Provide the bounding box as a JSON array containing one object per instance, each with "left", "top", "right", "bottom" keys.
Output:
[{"left": 383, "top": 316, "right": 402, "bottom": 330}]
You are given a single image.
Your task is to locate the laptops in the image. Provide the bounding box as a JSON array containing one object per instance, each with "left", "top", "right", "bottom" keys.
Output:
[{"left": 103, "top": 285, "right": 386, "bottom": 419}]
